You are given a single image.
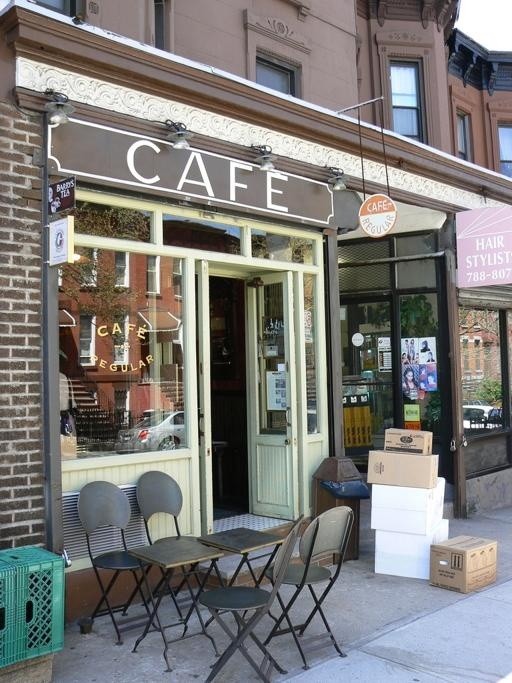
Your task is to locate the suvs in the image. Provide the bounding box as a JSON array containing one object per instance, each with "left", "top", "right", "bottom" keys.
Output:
[{"left": 462, "top": 399, "right": 502, "bottom": 429}]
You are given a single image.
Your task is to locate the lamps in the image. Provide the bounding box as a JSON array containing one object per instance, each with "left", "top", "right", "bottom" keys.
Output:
[
  {"left": 328, "top": 167, "right": 352, "bottom": 191},
  {"left": 165, "top": 118, "right": 194, "bottom": 149},
  {"left": 44, "top": 88, "right": 75, "bottom": 124},
  {"left": 251, "top": 143, "right": 281, "bottom": 171}
]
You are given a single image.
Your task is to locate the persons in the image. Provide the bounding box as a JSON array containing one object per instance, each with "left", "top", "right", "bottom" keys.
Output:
[{"left": 400, "top": 338, "right": 438, "bottom": 392}]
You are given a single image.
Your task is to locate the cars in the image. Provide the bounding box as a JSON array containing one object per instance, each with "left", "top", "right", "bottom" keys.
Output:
[{"left": 114, "top": 410, "right": 186, "bottom": 453}]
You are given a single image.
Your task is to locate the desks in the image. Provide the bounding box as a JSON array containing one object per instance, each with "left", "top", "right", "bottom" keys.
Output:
[
  {"left": 198, "top": 527, "right": 285, "bottom": 647},
  {"left": 127, "top": 539, "right": 224, "bottom": 672}
]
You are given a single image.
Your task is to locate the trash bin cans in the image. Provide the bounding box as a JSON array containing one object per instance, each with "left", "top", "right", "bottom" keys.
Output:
[{"left": 312, "top": 457, "right": 363, "bottom": 565}]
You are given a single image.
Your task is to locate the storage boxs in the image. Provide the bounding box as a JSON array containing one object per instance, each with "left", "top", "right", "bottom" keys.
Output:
[{"left": 367, "top": 428, "right": 497, "bottom": 594}]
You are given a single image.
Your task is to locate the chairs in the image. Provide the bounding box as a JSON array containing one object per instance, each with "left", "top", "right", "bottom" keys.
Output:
[
  {"left": 77, "top": 480, "right": 164, "bottom": 646},
  {"left": 135, "top": 471, "right": 203, "bottom": 623},
  {"left": 263, "top": 505, "right": 353, "bottom": 670},
  {"left": 200, "top": 514, "right": 304, "bottom": 682}
]
